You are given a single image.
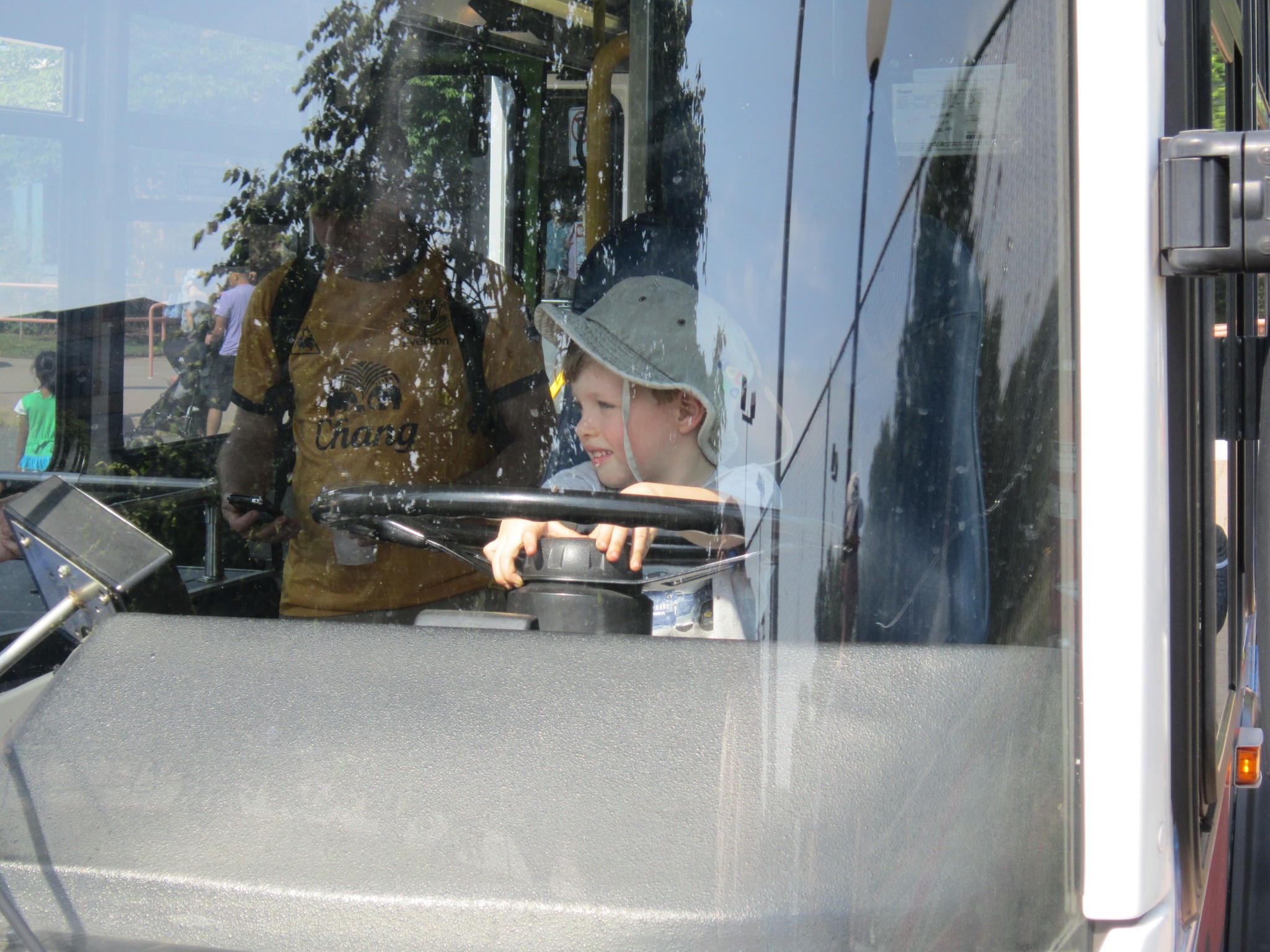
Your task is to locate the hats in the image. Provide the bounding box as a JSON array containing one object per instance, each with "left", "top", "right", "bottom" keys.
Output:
[{"left": 535, "top": 276, "right": 758, "bottom": 466}]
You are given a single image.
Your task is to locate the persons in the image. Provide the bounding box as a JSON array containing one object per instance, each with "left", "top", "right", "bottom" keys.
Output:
[
  {"left": 485, "top": 276, "right": 784, "bottom": 641},
  {"left": 15, "top": 349, "right": 56, "bottom": 478},
  {"left": 562, "top": 106, "right": 808, "bottom": 464},
  {"left": 164, "top": 268, "right": 257, "bottom": 436},
  {"left": 219, "top": 107, "right": 556, "bottom": 625}
]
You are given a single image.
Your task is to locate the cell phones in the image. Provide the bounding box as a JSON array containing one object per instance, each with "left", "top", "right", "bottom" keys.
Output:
[{"left": 226, "top": 493, "right": 282, "bottom": 525}]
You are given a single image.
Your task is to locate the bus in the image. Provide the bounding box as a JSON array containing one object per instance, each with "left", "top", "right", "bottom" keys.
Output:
[{"left": 0, "top": 0, "right": 1270, "bottom": 948}]
